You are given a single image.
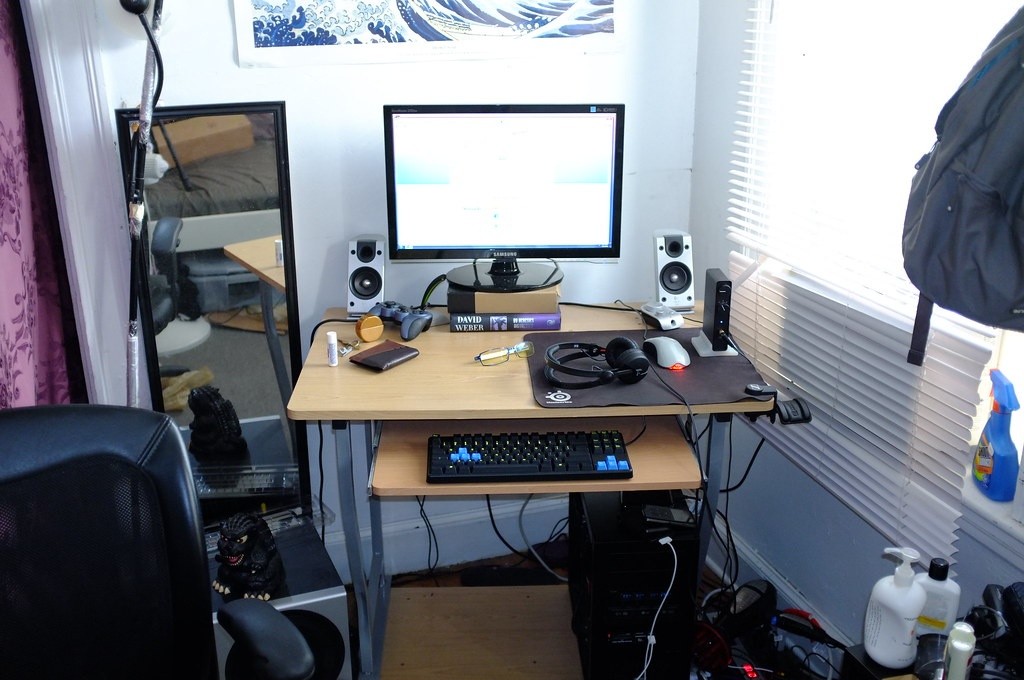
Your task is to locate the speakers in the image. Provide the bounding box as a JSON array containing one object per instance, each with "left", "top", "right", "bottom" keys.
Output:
[
  {"left": 347, "top": 234, "right": 386, "bottom": 320},
  {"left": 653, "top": 230, "right": 696, "bottom": 314},
  {"left": 207, "top": 513, "right": 356, "bottom": 679}
]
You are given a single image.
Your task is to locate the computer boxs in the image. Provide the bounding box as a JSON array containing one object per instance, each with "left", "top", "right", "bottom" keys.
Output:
[{"left": 567, "top": 493, "right": 696, "bottom": 680}]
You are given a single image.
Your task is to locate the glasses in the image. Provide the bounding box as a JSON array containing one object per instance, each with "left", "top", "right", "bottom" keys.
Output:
[{"left": 474, "top": 340, "right": 536, "bottom": 367}]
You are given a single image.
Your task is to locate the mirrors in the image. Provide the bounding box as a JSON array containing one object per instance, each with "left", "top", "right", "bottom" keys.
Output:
[{"left": 115, "top": 98, "right": 315, "bottom": 536}]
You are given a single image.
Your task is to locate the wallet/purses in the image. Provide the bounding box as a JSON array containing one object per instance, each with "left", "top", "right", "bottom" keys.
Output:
[{"left": 349, "top": 339, "right": 419, "bottom": 372}]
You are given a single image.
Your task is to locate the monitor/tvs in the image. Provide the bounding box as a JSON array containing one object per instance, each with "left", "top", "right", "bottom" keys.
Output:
[{"left": 381, "top": 103, "right": 625, "bottom": 263}]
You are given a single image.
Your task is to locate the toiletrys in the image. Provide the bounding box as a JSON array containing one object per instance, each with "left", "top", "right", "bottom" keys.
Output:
[
  {"left": 940, "top": 623, "right": 977, "bottom": 680},
  {"left": 864, "top": 546, "right": 927, "bottom": 671},
  {"left": 910, "top": 556, "right": 962, "bottom": 642}
]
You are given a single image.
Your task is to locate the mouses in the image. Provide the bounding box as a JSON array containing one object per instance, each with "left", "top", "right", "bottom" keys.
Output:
[{"left": 643, "top": 337, "right": 690, "bottom": 370}]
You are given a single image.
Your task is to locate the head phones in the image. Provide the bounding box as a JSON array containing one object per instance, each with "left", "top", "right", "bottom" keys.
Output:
[{"left": 544, "top": 336, "right": 650, "bottom": 389}]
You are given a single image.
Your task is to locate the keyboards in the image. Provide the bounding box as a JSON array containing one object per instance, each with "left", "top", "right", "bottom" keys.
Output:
[{"left": 426, "top": 429, "right": 633, "bottom": 485}]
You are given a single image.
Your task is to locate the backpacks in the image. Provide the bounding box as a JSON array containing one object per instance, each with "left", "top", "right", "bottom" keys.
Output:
[{"left": 901, "top": 5, "right": 1024, "bottom": 366}]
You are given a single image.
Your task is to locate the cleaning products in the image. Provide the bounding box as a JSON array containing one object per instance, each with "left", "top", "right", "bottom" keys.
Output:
[{"left": 970, "top": 366, "right": 1021, "bottom": 503}]
